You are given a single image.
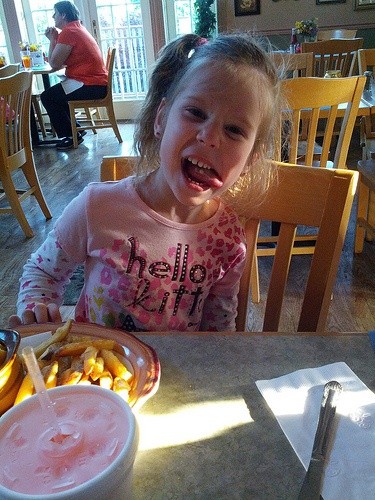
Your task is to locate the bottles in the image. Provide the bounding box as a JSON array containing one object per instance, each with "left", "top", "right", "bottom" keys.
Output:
[
  {"left": 324, "top": 70, "right": 341, "bottom": 78},
  {"left": 363, "top": 71, "right": 372, "bottom": 97},
  {"left": 288, "top": 28, "right": 303, "bottom": 54}
]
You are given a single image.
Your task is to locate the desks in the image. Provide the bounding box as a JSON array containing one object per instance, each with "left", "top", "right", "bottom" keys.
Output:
[
  {"left": 282, "top": 97, "right": 371, "bottom": 119},
  {"left": 20, "top": 61, "right": 67, "bottom": 145},
  {"left": 128, "top": 332, "right": 375, "bottom": 500},
  {"left": 361, "top": 83, "right": 375, "bottom": 135}
]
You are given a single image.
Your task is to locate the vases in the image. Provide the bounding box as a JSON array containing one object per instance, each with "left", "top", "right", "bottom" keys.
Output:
[{"left": 304, "top": 34, "right": 318, "bottom": 43}]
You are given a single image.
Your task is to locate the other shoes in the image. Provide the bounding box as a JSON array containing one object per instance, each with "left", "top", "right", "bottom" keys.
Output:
[{"left": 56, "top": 130, "right": 87, "bottom": 149}]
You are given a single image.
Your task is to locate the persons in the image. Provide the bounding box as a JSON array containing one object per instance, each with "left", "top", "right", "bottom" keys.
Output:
[
  {"left": 8, "top": 33, "right": 282, "bottom": 334},
  {"left": 40, "top": 1, "right": 109, "bottom": 149}
]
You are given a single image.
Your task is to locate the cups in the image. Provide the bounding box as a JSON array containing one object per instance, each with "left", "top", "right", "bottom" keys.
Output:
[
  {"left": 0, "top": 384, "right": 139, "bottom": 500},
  {"left": 20, "top": 51, "right": 32, "bottom": 69}
]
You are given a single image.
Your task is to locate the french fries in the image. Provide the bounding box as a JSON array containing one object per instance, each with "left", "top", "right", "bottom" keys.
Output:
[{"left": 0, "top": 319, "right": 133, "bottom": 419}]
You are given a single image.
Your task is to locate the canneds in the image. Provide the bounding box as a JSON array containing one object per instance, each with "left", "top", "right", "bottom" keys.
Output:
[{"left": 323, "top": 70, "right": 342, "bottom": 78}]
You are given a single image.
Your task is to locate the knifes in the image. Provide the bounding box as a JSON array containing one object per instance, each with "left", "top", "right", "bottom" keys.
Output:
[{"left": 294, "top": 381, "right": 340, "bottom": 500}]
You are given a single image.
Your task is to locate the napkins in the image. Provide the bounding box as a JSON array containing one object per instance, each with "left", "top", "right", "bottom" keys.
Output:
[{"left": 255, "top": 361, "right": 375, "bottom": 500}]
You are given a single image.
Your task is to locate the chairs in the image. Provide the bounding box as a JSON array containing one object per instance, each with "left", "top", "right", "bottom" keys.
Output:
[{"left": 0, "top": 29, "right": 375, "bottom": 333}]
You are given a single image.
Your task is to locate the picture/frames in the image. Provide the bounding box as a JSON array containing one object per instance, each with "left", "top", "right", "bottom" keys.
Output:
[
  {"left": 234, "top": 0, "right": 260, "bottom": 16},
  {"left": 354, "top": 0, "right": 375, "bottom": 11}
]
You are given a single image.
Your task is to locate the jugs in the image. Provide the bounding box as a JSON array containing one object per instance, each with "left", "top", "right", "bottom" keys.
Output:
[{"left": 0, "top": 328, "right": 23, "bottom": 416}]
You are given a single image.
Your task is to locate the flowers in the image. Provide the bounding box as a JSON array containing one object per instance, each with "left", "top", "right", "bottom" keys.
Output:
[
  {"left": 295, "top": 17, "right": 319, "bottom": 38},
  {"left": 19, "top": 41, "right": 39, "bottom": 52}
]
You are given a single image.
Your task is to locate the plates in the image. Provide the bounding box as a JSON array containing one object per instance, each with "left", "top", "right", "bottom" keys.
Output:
[{"left": 12, "top": 314, "right": 160, "bottom": 417}]
